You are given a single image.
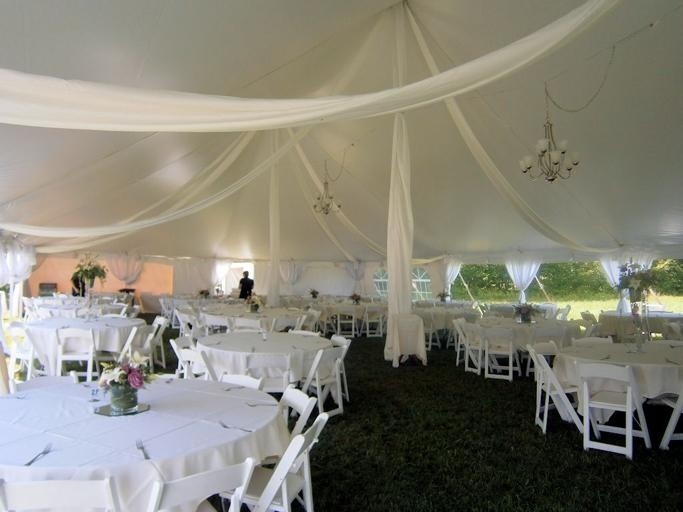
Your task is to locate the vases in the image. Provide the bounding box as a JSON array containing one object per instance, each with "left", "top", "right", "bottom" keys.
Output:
[
  {"left": 250, "top": 303, "right": 259, "bottom": 313},
  {"left": 352, "top": 300, "right": 359, "bottom": 305},
  {"left": 84, "top": 277, "right": 95, "bottom": 306},
  {"left": 109, "top": 386, "right": 139, "bottom": 412},
  {"left": 520, "top": 314, "right": 532, "bottom": 324},
  {"left": 629, "top": 287, "right": 650, "bottom": 351}
]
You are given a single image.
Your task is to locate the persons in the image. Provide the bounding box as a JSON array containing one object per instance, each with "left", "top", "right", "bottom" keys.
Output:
[
  {"left": 238, "top": 271, "right": 254, "bottom": 300},
  {"left": 70, "top": 271, "right": 86, "bottom": 298}
]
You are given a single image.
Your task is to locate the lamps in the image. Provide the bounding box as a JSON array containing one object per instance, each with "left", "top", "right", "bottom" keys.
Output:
[
  {"left": 312, "top": 158, "right": 343, "bottom": 215},
  {"left": 518, "top": 81, "right": 582, "bottom": 184}
]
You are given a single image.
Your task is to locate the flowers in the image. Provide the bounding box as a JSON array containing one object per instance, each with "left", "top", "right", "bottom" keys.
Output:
[
  {"left": 70, "top": 251, "right": 106, "bottom": 289},
  {"left": 512, "top": 303, "right": 542, "bottom": 325},
  {"left": 607, "top": 257, "right": 662, "bottom": 346},
  {"left": 99, "top": 351, "right": 159, "bottom": 409},
  {"left": 247, "top": 295, "right": 263, "bottom": 309},
  {"left": 436, "top": 288, "right": 451, "bottom": 303},
  {"left": 349, "top": 293, "right": 361, "bottom": 305}
]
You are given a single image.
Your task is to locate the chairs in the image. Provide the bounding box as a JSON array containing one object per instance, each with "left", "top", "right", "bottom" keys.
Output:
[
  {"left": 337, "top": 304, "right": 359, "bottom": 338},
  {"left": 54, "top": 326, "right": 101, "bottom": 382},
  {"left": 360, "top": 303, "right": 383, "bottom": 338},
  {"left": 113, "top": 325, "right": 155, "bottom": 374},
  {"left": 52, "top": 291, "right": 61, "bottom": 297},
  {"left": 218, "top": 373, "right": 266, "bottom": 391},
  {"left": 233, "top": 316, "right": 264, "bottom": 330},
  {"left": 131, "top": 304, "right": 140, "bottom": 319},
  {"left": 302, "top": 307, "right": 322, "bottom": 331},
  {"left": 101, "top": 296, "right": 113, "bottom": 300},
  {"left": 289, "top": 329, "right": 322, "bottom": 337},
  {"left": 172, "top": 307, "right": 205, "bottom": 340},
  {"left": 270, "top": 315, "right": 301, "bottom": 331},
  {"left": 581, "top": 309, "right": 599, "bottom": 338},
  {"left": 554, "top": 304, "right": 572, "bottom": 321},
  {"left": 176, "top": 348, "right": 218, "bottom": 382},
  {"left": 158, "top": 295, "right": 174, "bottom": 321},
  {"left": 645, "top": 303, "right": 665, "bottom": 312},
  {"left": 446, "top": 327, "right": 455, "bottom": 349},
  {"left": 570, "top": 335, "right": 615, "bottom": 347},
  {"left": 146, "top": 455, "right": 258, "bottom": 512},
  {"left": 219, "top": 412, "right": 330, "bottom": 512},
  {"left": 362, "top": 296, "right": 372, "bottom": 303},
  {"left": 574, "top": 361, "right": 652, "bottom": 460},
  {"left": 4, "top": 324, "right": 48, "bottom": 382},
  {"left": 535, "top": 352, "right": 585, "bottom": 435},
  {"left": 659, "top": 395, "right": 683, "bottom": 450},
  {"left": 330, "top": 334, "right": 352, "bottom": 402},
  {"left": 296, "top": 306, "right": 312, "bottom": 330},
  {"left": 488, "top": 302, "right": 516, "bottom": 318},
  {"left": 453, "top": 320, "right": 479, "bottom": 369},
  {"left": 570, "top": 317, "right": 596, "bottom": 338},
  {"left": 462, "top": 323, "right": 487, "bottom": 375},
  {"left": 290, "top": 345, "right": 348, "bottom": 418},
  {"left": 311, "top": 303, "right": 337, "bottom": 335},
  {"left": 278, "top": 382, "right": 318, "bottom": 440},
  {"left": 454, "top": 317, "right": 467, "bottom": 352},
  {"left": 147, "top": 315, "right": 169, "bottom": 368},
  {"left": 412, "top": 306, "right": 441, "bottom": 351},
  {"left": 99, "top": 303, "right": 128, "bottom": 318},
  {"left": 373, "top": 295, "right": 384, "bottom": 303},
  {"left": 526, "top": 340, "right": 557, "bottom": 382},
  {"left": 0, "top": 475, "right": 124, "bottom": 510},
  {"left": 24, "top": 306, "right": 34, "bottom": 319},
  {"left": 519, "top": 323, "right": 568, "bottom": 377},
  {"left": 169, "top": 335, "right": 197, "bottom": 377},
  {"left": 202, "top": 313, "right": 231, "bottom": 336},
  {"left": 541, "top": 302, "right": 559, "bottom": 320},
  {"left": 20, "top": 296, "right": 33, "bottom": 308},
  {"left": 485, "top": 325, "right": 522, "bottom": 381}
]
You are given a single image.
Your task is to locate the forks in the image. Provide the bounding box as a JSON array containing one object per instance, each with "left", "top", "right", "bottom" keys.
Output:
[
  {"left": 221, "top": 385, "right": 249, "bottom": 391},
  {"left": 3, "top": 393, "right": 29, "bottom": 401},
  {"left": 217, "top": 420, "right": 252, "bottom": 436},
  {"left": 134, "top": 441, "right": 150, "bottom": 463},
  {"left": 247, "top": 397, "right": 279, "bottom": 410},
  {"left": 26, "top": 443, "right": 55, "bottom": 466}
]
